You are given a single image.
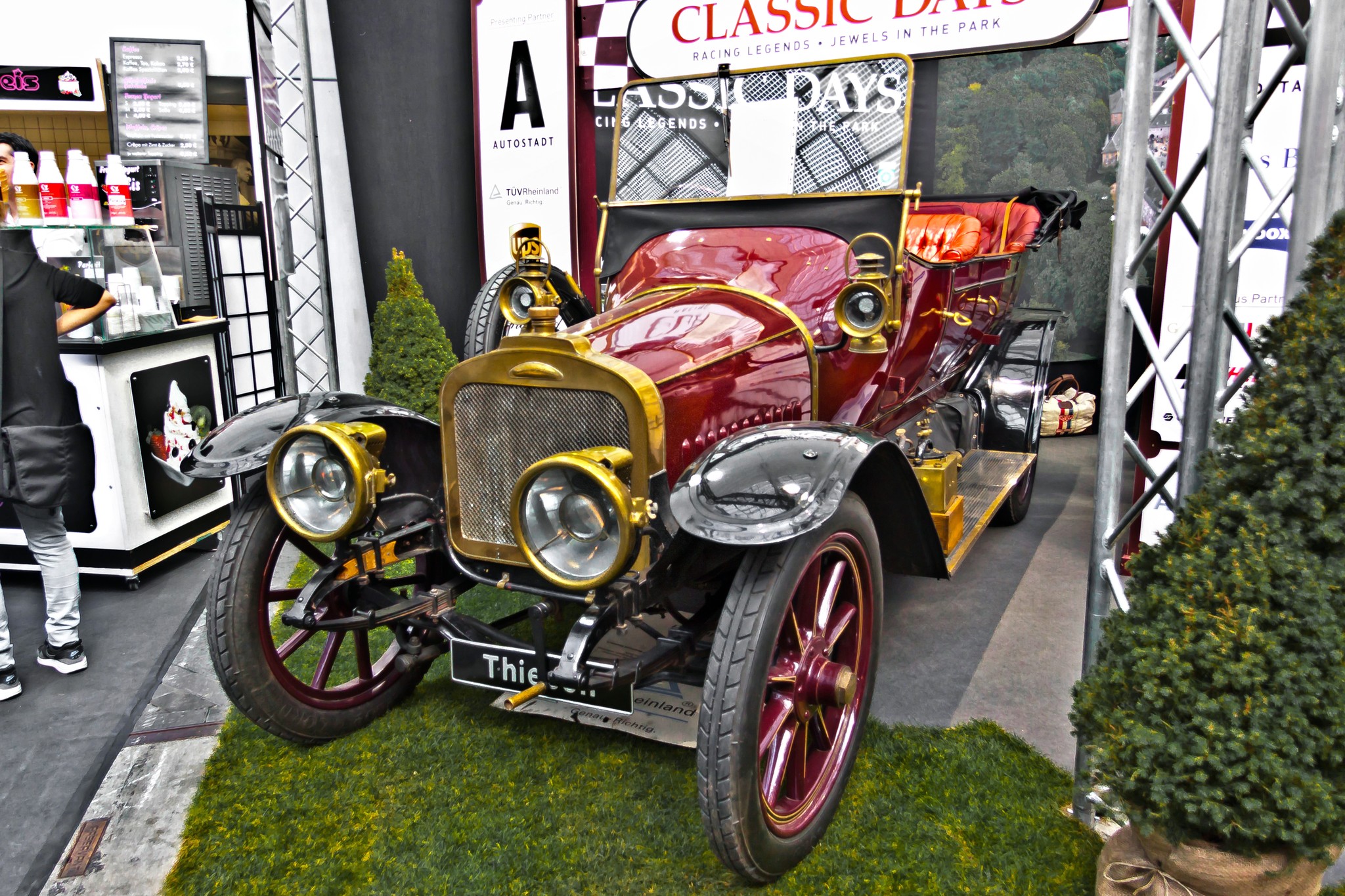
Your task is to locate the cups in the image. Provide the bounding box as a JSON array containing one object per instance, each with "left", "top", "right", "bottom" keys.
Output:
[{"left": 103, "top": 267, "right": 186, "bottom": 336}]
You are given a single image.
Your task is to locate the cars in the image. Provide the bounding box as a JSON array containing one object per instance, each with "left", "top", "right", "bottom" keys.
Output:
[{"left": 177, "top": 52, "right": 1088, "bottom": 887}]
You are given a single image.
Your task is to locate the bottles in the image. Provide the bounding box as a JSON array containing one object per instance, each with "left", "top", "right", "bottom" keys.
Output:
[
  {"left": 11, "top": 151, "right": 45, "bottom": 227},
  {"left": 105, "top": 154, "right": 135, "bottom": 226},
  {"left": 82, "top": 155, "right": 103, "bottom": 226},
  {"left": 66, "top": 150, "right": 99, "bottom": 226},
  {"left": 38, "top": 150, "right": 70, "bottom": 227}
]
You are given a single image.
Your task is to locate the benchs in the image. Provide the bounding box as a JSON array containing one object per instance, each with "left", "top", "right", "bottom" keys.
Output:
[{"left": 904, "top": 200, "right": 1040, "bottom": 256}]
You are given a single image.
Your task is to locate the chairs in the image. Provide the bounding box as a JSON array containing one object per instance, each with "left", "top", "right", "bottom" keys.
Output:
[{"left": 907, "top": 209, "right": 983, "bottom": 262}]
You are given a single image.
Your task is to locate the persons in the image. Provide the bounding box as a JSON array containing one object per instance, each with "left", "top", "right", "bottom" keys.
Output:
[
  {"left": 0, "top": 132, "right": 40, "bottom": 189},
  {"left": 0, "top": 190, "right": 118, "bottom": 701}
]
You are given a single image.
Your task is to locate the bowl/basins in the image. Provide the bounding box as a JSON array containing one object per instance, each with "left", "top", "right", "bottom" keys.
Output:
[{"left": 151, "top": 451, "right": 195, "bottom": 487}]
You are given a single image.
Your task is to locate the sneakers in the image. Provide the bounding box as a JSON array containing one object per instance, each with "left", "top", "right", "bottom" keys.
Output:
[
  {"left": 0, "top": 661, "right": 24, "bottom": 703},
  {"left": 37, "top": 637, "right": 87, "bottom": 676}
]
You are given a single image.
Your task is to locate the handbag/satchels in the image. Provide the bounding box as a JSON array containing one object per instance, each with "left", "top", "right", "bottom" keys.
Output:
[{"left": 0, "top": 423, "right": 95, "bottom": 511}]
